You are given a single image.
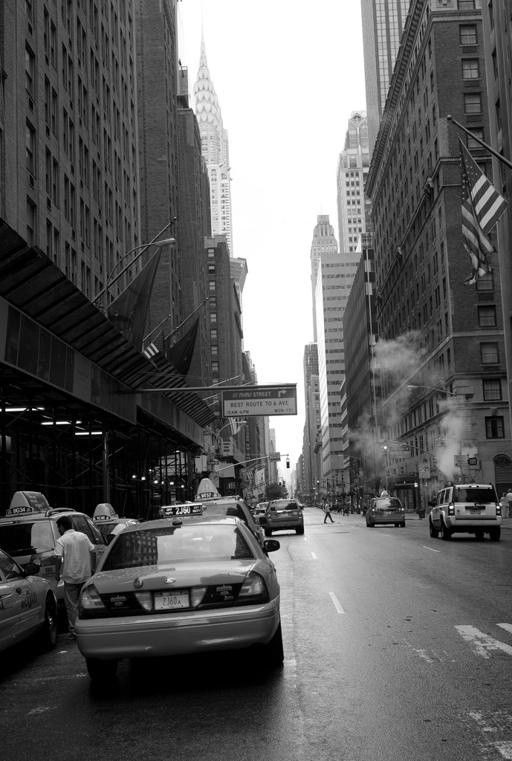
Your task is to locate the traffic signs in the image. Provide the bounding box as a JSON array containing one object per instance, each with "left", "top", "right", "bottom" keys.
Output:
[{"left": 220, "top": 385, "right": 297, "bottom": 418}]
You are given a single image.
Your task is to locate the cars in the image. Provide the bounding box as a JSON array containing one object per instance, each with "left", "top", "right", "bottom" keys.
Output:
[
  {"left": 74, "top": 504, "right": 285, "bottom": 681},
  {"left": 365, "top": 491, "right": 406, "bottom": 530},
  {"left": 164, "top": 479, "right": 265, "bottom": 553},
  {"left": 87, "top": 501, "right": 143, "bottom": 547},
  {"left": 0, "top": 550, "right": 59, "bottom": 668}
]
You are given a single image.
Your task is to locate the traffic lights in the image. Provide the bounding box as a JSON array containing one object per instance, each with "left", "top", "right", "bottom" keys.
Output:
[
  {"left": 383, "top": 444, "right": 388, "bottom": 451},
  {"left": 286, "top": 461, "right": 289, "bottom": 468},
  {"left": 317, "top": 480, "right": 319, "bottom": 485}
]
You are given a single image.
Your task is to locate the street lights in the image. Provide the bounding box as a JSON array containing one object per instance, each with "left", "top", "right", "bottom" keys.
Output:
[
  {"left": 213, "top": 418, "right": 247, "bottom": 448},
  {"left": 406, "top": 385, "right": 462, "bottom": 485}
]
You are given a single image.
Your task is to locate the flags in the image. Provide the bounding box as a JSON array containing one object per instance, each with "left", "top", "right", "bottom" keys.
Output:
[{"left": 458, "top": 133, "right": 510, "bottom": 287}]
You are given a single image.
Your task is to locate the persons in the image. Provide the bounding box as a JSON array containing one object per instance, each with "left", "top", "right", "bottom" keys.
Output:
[
  {"left": 323, "top": 495, "right": 362, "bottom": 524},
  {"left": 506, "top": 489, "right": 512, "bottom": 517},
  {"left": 500, "top": 493, "right": 509, "bottom": 519},
  {"left": 50, "top": 517, "right": 98, "bottom": 640}
]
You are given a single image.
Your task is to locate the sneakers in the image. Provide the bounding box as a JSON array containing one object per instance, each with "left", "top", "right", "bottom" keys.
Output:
[{"left": 67, "top": 634, "right": 75, "bottom": 639}]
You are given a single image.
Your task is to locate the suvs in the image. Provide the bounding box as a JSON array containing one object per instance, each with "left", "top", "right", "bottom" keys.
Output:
[
  {"left": 253, "top": 498, "right": 305, "bottom": 539},
  {"left": 1, "top": 491, "right": 111, "bottom": 633},
  {"left": 428, "top": 485, "right": 502, "bottom": 542}
]
